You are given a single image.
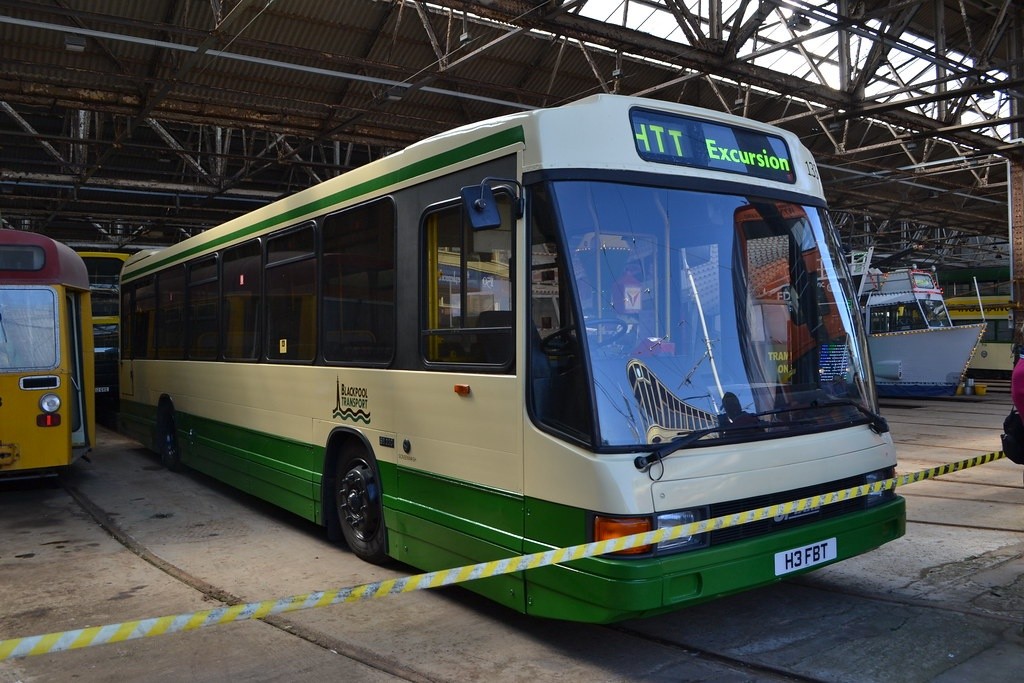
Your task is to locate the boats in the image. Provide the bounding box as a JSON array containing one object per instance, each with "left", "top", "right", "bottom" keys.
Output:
[{"left": 820, "top": 251, "right": 985, "bottom": 399}]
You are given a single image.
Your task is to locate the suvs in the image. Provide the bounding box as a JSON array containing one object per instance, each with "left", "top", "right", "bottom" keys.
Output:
[{"left": 1001, "top": 347, "right": 1024, "bottom": 465}]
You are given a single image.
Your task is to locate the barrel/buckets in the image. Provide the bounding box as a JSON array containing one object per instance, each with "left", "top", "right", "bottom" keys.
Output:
[{"left": 956, "top": 378, "right": 987, "bottom": 395}]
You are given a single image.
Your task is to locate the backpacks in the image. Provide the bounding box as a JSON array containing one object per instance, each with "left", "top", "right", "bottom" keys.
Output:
[{"left": 1000, "top": 405, "right": 1024, "bottom": 465}]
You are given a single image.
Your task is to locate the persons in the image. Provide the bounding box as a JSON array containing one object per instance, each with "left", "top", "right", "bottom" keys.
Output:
[
  {"left": 1011, "top": 317, "right": 1024, "bottom": 424},
  {"left": 899, "top": 317, "right": 913, "bottom": 330}
]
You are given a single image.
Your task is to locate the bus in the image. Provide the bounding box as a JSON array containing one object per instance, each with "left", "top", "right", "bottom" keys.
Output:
[
  {"left": 74, "top": 251, "right": 133, "bottom": 418},
  {"left": 897, "top": 295, "right": 1012, "bottom": 380},
  {"left": 0, "top": 228, "right": 95, "bottom": 482},
  {"left": 121, "top": 93, "right": 908, "bottom": 618}
]
